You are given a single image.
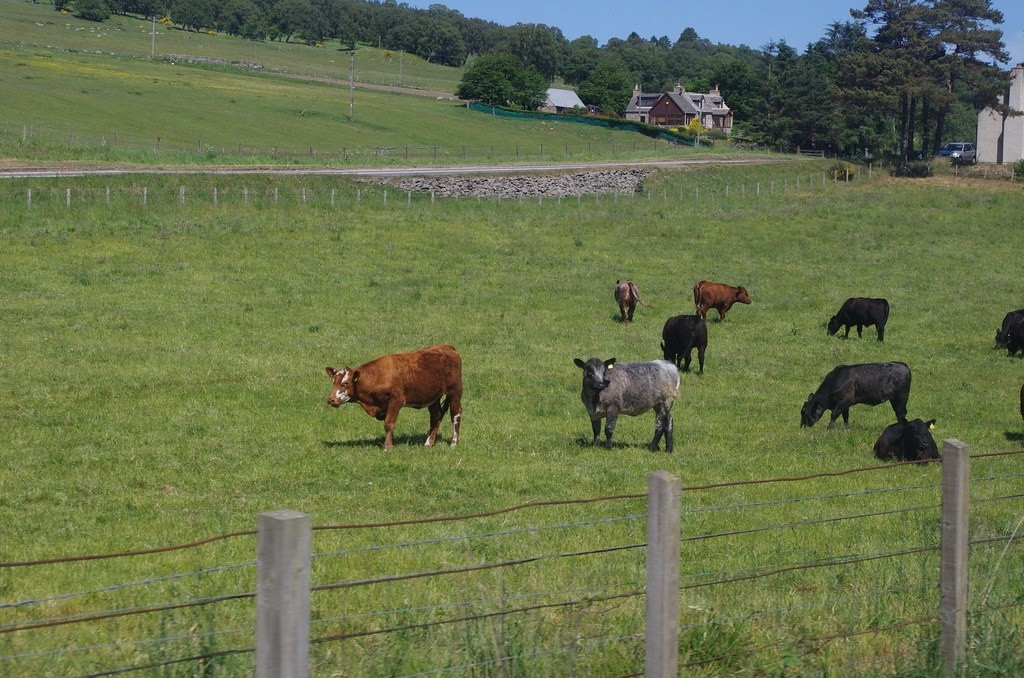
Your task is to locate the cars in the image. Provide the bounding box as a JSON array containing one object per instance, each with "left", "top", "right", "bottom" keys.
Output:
[{"left": 588, "top": 106, "right": 604, "bottom": 113}]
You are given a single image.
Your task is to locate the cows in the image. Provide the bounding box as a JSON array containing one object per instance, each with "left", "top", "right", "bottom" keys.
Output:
[
  {"left": 660, "top": 314, "right": 708, "bottom": 374},
  {"left": 994, "top": 309, "right": 1024, "bottom": 357},
  {"left": 614, "top": 278, "right": 652, "bottom": 325},
  {"left": 801, "top": 361, "right": 912, "bottom": 430},
  {"left": 325, "top": 343, "right": 464, "bottom": 450},
  {"left": 694, "top": 277, "right": 753, "bottom": 321},
  {"left": 827, "top": 297, "right": 890, "bottom": 340},
  {"left": 573, "top": 357, "right": 682, "bottom": 450},
  {"left": 873, "top": 418, "right": 942, "bottom": 466}
]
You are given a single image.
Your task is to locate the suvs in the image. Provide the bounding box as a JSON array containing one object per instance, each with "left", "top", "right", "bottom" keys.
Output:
[{"left": 940, "top": 142, "right": 977, "bottom": 164}]
